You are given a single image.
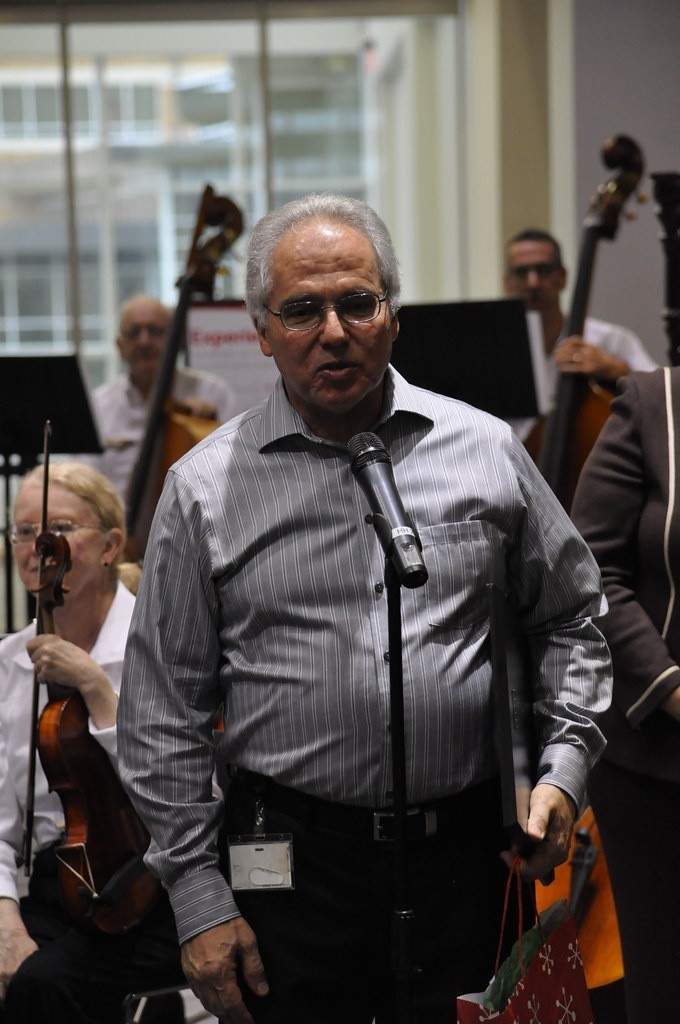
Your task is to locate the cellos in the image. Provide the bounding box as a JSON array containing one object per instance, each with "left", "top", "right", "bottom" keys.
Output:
[
  {"left": 535, "top": 802, "right": 625, "bottom": 992},
  {"left": 523, "top": 130, "right": 649, "bottom": 512},
  {"left": 119, "top": 181, "right": 243, "bottom": 566}
]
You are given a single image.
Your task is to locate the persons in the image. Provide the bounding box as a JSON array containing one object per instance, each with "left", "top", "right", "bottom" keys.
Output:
[
  {"left": 487, "top": 229, "right": 664, "bottom": 448},
  {"left": 114, "top": 194, "right": 614, "bottom": 1024},
  {"left": 0, "top": 463, "right": 200, "bottom": 1024},
  {"left": 36, "top": 295, "right": 232, "bottom": 532},
  {"left": 566, "top": 364, "right": 679, "bottom": 1024}
]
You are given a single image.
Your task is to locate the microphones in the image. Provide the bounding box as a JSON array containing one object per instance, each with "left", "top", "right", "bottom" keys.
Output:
[{"left": 346, "top": 432, "right": 429, "bottom": 590}]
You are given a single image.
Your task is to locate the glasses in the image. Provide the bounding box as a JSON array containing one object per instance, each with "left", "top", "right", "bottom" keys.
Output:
[
  {"left": 3, "top": 518, "right": 107, "bottom": 547},
  {"left": 262, "top": 288, "right": 390, "bottom": 332},
  {"left": 508, "top": 261, "right": 561, "bottom": 280}
]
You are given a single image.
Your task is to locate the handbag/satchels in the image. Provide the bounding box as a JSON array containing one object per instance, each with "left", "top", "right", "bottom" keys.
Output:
[{"left": 457, "top": 866, "right": 590, "bottom": 1024}]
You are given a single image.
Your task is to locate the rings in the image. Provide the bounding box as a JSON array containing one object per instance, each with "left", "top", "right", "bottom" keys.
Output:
[{"left": 572, "top": 352, "right": 582, "bottom": 363}]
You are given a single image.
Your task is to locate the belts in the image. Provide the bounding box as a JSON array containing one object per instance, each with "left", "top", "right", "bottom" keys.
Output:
[{"left": 219, "top": 766, "right": 500, "bottom": 845}]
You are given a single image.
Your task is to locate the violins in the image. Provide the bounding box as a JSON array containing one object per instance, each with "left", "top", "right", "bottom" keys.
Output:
[{"left": 21, "top": 409, "right": 161, "bottom": 935}]
